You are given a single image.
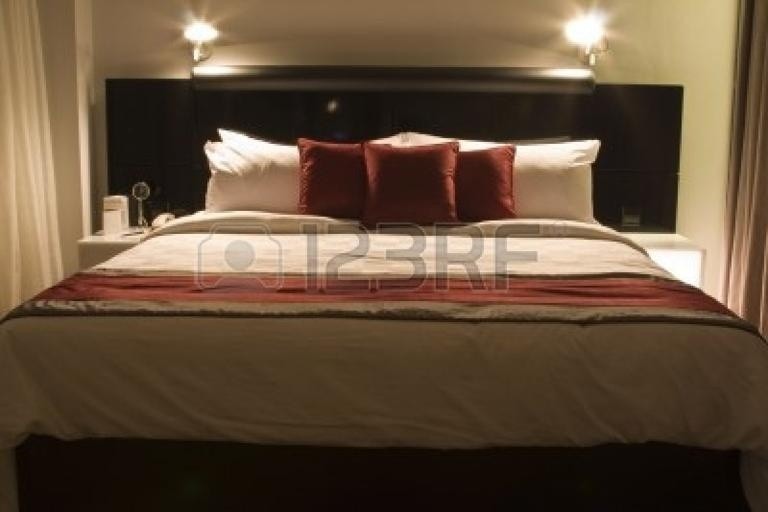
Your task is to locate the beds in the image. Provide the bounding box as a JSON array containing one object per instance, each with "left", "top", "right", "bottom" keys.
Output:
[{"left": 0, "top": 70, "right": 768, "bottom": 512}]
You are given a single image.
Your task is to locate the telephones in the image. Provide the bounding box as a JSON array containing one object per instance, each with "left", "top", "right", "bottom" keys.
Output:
[{"left": 151, "top": 213, "right": 175, "bottom": 231}]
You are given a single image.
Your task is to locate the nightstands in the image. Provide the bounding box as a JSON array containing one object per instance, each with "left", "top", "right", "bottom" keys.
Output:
[
  {"left": 621, "top": 232, "right": 706, "bottom": 290},
  {"left": 76, "top": 227, "right": 152, "bottom": 271}
]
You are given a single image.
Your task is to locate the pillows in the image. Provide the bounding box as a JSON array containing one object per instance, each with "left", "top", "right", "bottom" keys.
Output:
[{"left": 203, "top": 127, "right": 602, "bottom": 229}]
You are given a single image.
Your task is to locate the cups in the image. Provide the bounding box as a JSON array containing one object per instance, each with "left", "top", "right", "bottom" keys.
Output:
[{"left": 103, "top": 209, "right": 121, "bottom": 235}]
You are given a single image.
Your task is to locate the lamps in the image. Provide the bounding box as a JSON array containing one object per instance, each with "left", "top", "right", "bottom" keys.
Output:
[{"left": 132, "top": 182, "right": 151, "bottom": 234}]
